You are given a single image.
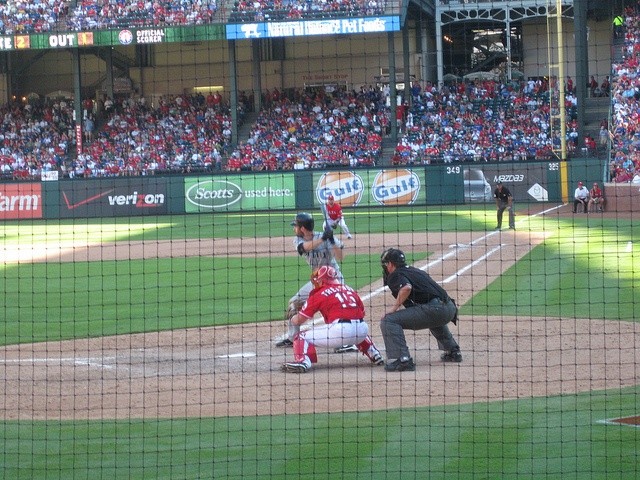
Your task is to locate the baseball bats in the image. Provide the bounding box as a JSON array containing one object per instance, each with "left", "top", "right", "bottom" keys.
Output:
[{"left": 321, "top": 202, "right": 327, "bottom": 226}]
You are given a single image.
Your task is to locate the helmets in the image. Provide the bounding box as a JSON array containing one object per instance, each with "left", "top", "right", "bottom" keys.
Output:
[
  {"left": 310, "top": 266, "right": 340, "bottom": 287},
  {"left": 381, "top": 247, "right": 406, "bottom": 286}
]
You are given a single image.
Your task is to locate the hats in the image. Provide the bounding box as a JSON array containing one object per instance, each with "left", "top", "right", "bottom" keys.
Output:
[
  {"left": 577, "top": 182, "right": 583, "bottom": 186},
  {"left": 327, "top": 195, "right": 334, "bottom": 201},
  {"left": 592, "top": 182, "right": 598, "bottom": 186},
  {"left": 496, "top": 180, "right": 502, "bottom": 186},
  {"left": 290, "top": 212, "right": 314, "bottom": 232}
]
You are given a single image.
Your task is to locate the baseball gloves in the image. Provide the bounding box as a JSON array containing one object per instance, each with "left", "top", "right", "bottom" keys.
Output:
[{"left": 287, "top": 299, "right": 304, "bottom": 320}]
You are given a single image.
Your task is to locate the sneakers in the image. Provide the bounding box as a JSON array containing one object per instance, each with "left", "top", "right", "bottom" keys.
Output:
[
  {"left": 441, "top": 350, "right": 463, "bottom": 363},
  {"left": 384, "top": 358, "right": 415, "bottom": 372},
  {"left": 347, "top": 235, "right": 352, "bottom": 239},
  {"left": 598, "top": 207, "right": 603, "bottom": 211},
  {"left": 334, "top": 344, "right": 359, "bottom": 354},
  {"left": 371, "top": 354, "right": 382, "bottom": 364},
  {"left": 276, "top": 338, "right": 294, "bottom": 348},
  {"left": 280, "top": 362, "right": 308, "bottom": 372}
]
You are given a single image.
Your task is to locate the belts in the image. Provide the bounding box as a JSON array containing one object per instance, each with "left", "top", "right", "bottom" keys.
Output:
[
  {"left": 333, "top": 318, "right": 364, "bottom": 324},
  {"left": 433, "top": 295, "right": 450, "bottom": 303}
]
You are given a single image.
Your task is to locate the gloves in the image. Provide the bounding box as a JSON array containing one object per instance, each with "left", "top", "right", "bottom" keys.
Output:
[
  {"left": 324, "top": 227, "right": 335, "bottom": 244},
  {"left": 321, "top": 230, "right": 333, "bottom": 240}
]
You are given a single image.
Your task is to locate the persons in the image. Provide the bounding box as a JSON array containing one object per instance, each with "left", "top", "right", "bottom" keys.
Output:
[
  {"left": 587, "top": 76, "right": 611, "bottom": 96},
  {"left": 584, "top": 133, "right": 595, "bottom": 152},
  {"left": 494, "top": 181, "right": 515, "bottom": 229},
  {"left": 274, "top": 213, "right": 358, "bottom": 353},
  {"left": 1, "top": 2, "right": 389, "bottom": 34},
  {"left": 608, "top": 0, "right": 640, "bottom": 183},
  {"left": 588, "top": 183, "right": 605, "bottom": 213},
  {"left": 322, "top": 192, "right": 356, "bottom": 240},
  {"left": 1, "top": 76, "right": 580, "bottom": 180},
  {"left": 573, "top": 181, "right": 589, "bottom": 214},
  {"left": 276, "top": 265, "right": 384, "bottom": 375},
  {"left": 599, "top": 116, "right": 610, "bottom": 154},
  {"left": 380, "top": 247, "right": 469, "bottom": 371}
]
